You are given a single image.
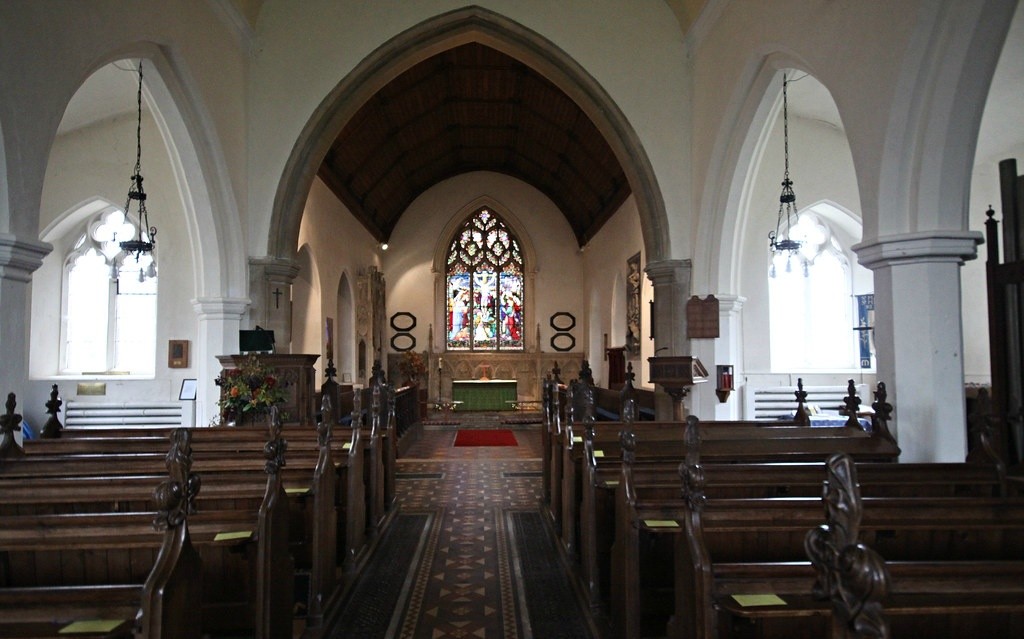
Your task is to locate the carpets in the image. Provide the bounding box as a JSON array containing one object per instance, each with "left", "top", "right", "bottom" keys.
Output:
[{"left": 453, "top": 428, "right": 519, "bottom": 448}]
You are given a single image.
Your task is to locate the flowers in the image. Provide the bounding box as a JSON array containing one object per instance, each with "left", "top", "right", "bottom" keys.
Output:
[
  {"left": 397, "top": 350, "right": 426, "bottom": 384},
  {"left": 213, "top": 351, "right": 290, "bottom": 426}
]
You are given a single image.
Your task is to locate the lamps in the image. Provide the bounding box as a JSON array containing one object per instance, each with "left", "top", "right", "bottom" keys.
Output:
[
  {"left": 101, "top": 57, "right": 160, "bottom": 285},
  {"left": 764, "top": 72, "right": 815, "bottom": 280}
]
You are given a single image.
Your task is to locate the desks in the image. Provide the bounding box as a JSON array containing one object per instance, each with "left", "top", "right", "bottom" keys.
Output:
[{"left": 452, "top": 379, "right": 518, "bottom": 410}]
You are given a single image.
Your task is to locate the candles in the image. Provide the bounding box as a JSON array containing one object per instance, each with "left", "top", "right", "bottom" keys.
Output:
[{"left": 439, "top": 358, "right": 443, "bottom": 369}]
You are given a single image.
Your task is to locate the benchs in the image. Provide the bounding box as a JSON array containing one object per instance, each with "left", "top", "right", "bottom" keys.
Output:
[
  {"left": 0, "top": 378, "right": 400, "bottom": 638},
  {"left": 537, "top": 359, "right": 1024, "bottom": 639}
]
